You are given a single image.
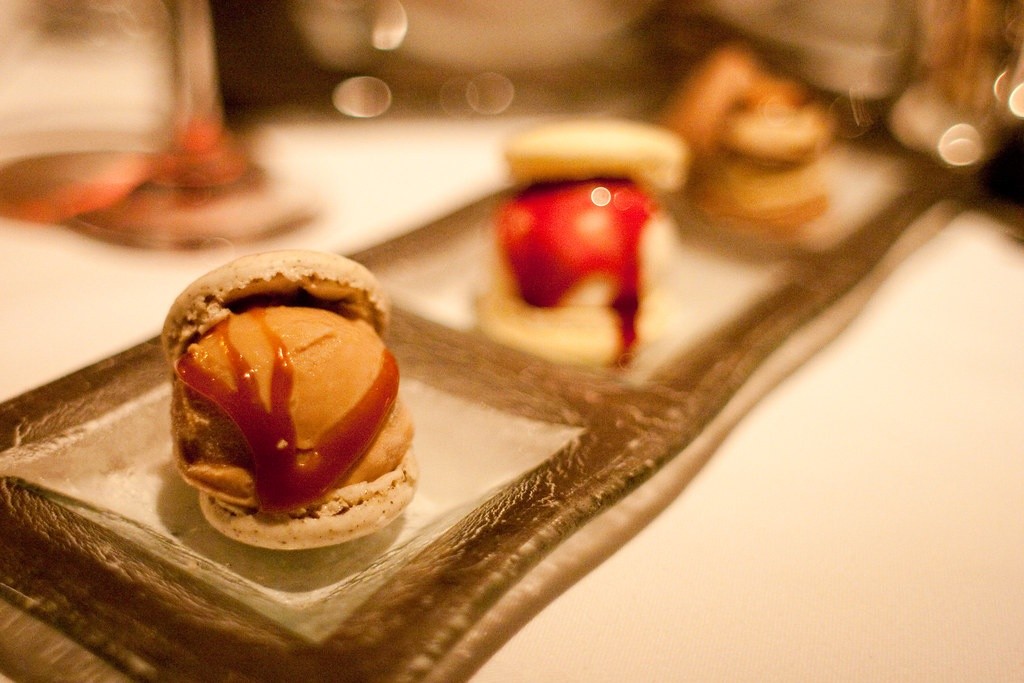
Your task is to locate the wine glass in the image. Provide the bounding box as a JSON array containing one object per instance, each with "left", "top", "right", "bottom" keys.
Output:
[{"left": 72, "top": 0, "right": 311, "bottom": 246}]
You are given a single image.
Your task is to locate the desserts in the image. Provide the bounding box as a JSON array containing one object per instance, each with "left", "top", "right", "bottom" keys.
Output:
[
  {"left": 659, "top": 44, "right": 842, "bottom": 259},
  {"left": 157, "top": 244, "right": 419, "bottom": 549},
  {"left": 475, "top": 110, "right": 690, "bottom": 371}
]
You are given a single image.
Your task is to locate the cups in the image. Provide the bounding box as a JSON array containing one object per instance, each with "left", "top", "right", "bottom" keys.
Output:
[
  {"left": 296, "top": 0, "right": 668, "bottom": 116},
  {"left": 0, "top": 0, "right": 172, "bottom": 221}
]
